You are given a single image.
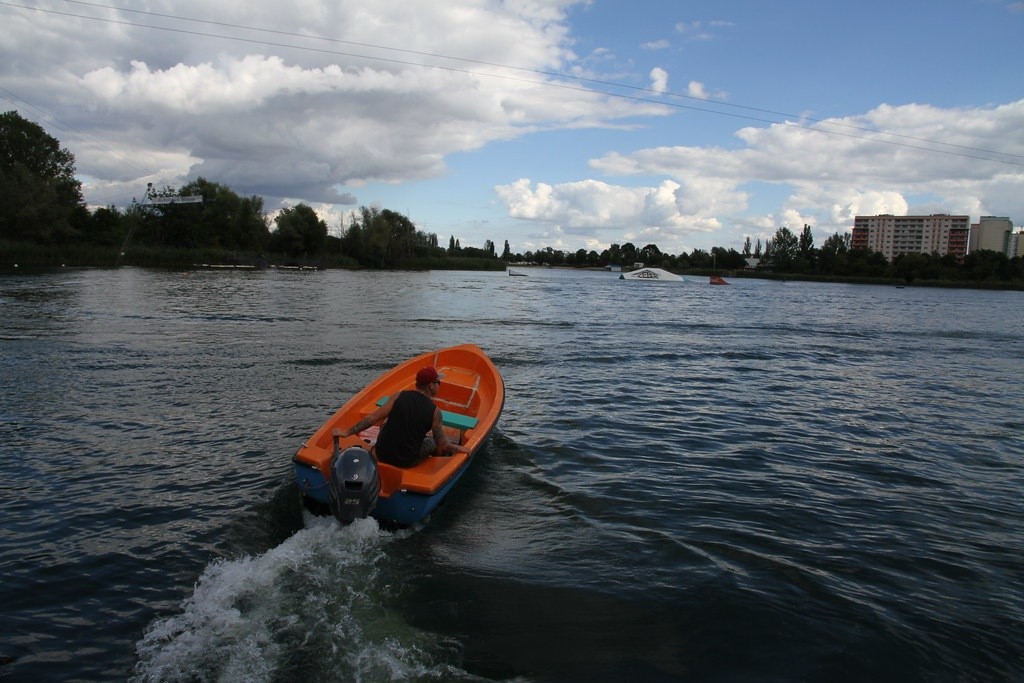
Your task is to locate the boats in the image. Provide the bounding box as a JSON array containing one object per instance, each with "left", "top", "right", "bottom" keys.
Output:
[
  {"left": 290, "top": 342, "right": 506, "bottom": 532},
  {"left": 708, "top": 275, "right": 731, "bottom": 286}
]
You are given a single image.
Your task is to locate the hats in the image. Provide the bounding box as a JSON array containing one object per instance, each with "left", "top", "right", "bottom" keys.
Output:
[{"left": 416, "top": 367, "right": 438, "bottom": 384}]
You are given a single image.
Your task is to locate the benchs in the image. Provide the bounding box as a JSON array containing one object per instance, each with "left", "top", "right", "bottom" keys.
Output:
[{"left": 378, "top": 396, "right": 479, "bottom": 428}]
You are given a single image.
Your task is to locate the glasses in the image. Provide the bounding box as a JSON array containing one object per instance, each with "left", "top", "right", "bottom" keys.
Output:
[{"left": 432, "top": 381, "right": 440, "bottom": 386}]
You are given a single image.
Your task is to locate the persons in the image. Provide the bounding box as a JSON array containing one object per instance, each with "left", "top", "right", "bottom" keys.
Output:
[{"left": 329, "top": 365, "right": 470, "bottom": 468}]
